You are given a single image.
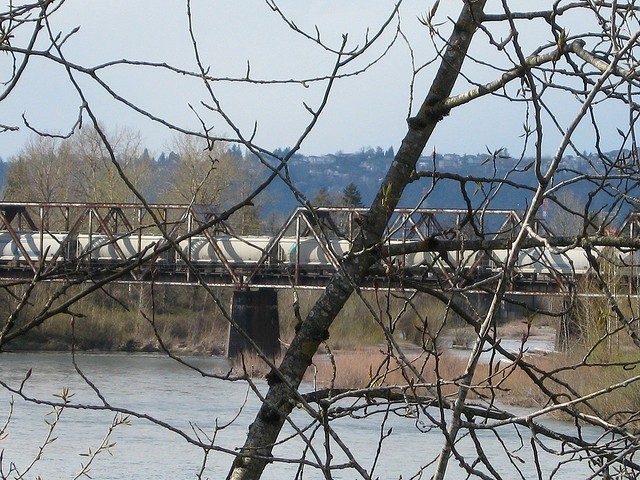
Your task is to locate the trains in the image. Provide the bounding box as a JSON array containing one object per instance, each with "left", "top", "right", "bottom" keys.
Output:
[{"left": 0, "top": 229, "right": 640, "bottom": 282}]
